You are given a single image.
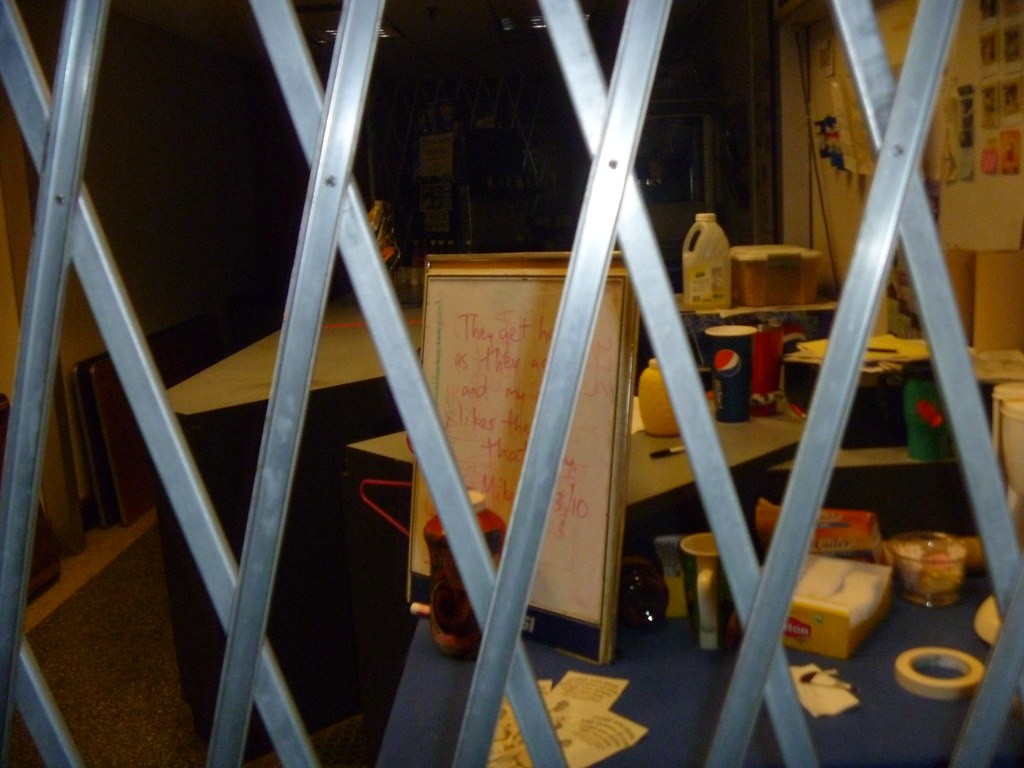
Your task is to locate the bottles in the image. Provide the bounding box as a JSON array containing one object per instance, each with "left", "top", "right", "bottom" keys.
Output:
[
  {"left": 425, "top": 492, "right": 507, "bottom": 655},
  {"left": 683, "top": 214, "right": 733, "bottom": 310},
  {"left": 638, "top": 359, "right": 681, "bottom": 436}
]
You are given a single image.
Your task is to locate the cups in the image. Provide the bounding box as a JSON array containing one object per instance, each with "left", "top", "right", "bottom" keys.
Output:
[
  {"left": 680, "top": 534, "right": 734, "bottom": 650},
  {"left": 890, "top": 531, "right": 969, "bottom": 605},
  {"left": 902, "top": 359, "right": 946, "bottom": 462},
  {"left": 706, "top": 327, "right": 758, "bottom": 423},
  {"left": 748, "top": 325, "right": 786, "bottom": 416}
]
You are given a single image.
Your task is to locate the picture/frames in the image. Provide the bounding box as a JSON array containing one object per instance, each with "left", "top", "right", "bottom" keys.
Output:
[{"left": 635, "top": 114, "right": 705, "bottom": 205}]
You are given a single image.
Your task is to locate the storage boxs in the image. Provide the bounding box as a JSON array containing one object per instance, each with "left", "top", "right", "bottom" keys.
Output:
[
  {"left": 783, "top": 555, "right": 895, "bottom": 660},
  {"left": 730, "top": 245, "right": 824, "bottom": 307}
]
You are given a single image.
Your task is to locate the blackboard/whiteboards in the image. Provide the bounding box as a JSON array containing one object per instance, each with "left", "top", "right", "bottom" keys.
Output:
[{"left": 406, "top": 249, "right": 639, "bottom": 630}]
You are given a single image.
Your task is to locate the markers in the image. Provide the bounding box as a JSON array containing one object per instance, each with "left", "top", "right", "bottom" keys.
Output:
[{"left": 410, "top": 601, "right": 431, "bottom": 616}]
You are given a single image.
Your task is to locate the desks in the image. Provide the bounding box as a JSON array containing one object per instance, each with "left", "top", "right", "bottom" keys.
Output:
[
  {"left": 672, "top": 294, "right": 838, "bottom": 326},
  {"left": 758, "top": 444, "right": 960, "bottom": 539},
  {"left": 375, "top": 574, "right": 1024, "bottom": 768},
  {"left": 153, "top": 300, "right": 423, "bottom": 766},
  {"left": 345, "top": 393, "right": 809, "bottom": 768}
]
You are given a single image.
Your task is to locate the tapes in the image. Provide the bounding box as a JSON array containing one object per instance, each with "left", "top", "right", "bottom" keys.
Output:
[{"left": 894, "top": 646, "right": 986, "bottom": 701}]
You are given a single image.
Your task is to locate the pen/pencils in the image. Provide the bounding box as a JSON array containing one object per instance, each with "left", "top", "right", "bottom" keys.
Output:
[
  {"left": 650, "top": 446, "right": 686, "bottom": 460},
  {"left": 866, "top": 347, "right": 896, "bottom": 353},
  {"left": 789, "top": 401, "right": 806, "bottom": 418}
]
